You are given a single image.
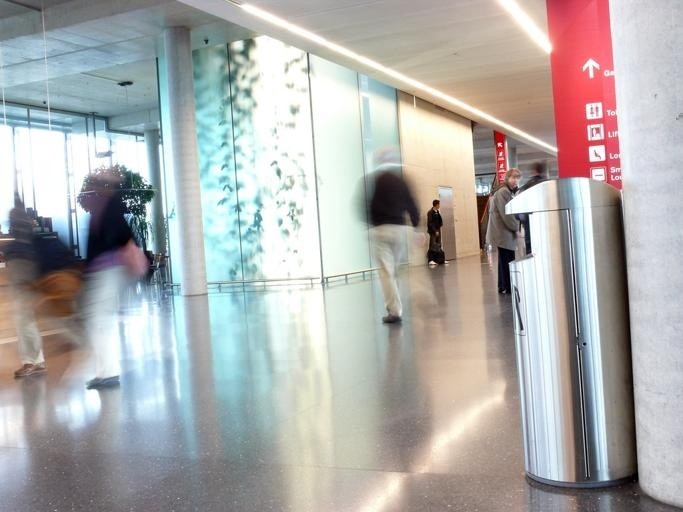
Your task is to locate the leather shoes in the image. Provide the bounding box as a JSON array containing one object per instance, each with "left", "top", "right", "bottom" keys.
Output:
[{"left": 87, "top": 377, "right": 119, "bottom": 386}]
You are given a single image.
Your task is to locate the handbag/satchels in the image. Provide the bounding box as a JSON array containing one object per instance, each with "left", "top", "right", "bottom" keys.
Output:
[{"left": 428, "top": 242, "right": 445, "bottom": 262}]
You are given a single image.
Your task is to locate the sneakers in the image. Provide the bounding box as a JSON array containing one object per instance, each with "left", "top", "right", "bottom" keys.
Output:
[
  {"left": 15, "top": 363, "right": 46, "bottom": 377},
  {"left": 428, "top": 261, "right": 437, "bottom": 265},
  {"left": 383, "top": 314, "right": 401, "bottom": 324},
  {"left": 444, "top": 261, "right": 448, "bottom": 264},
  {"left": 499, "top": 288, "right": 510, "bottom": 294}
]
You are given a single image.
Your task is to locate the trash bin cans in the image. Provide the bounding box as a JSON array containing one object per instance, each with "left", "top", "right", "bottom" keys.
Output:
[{"left": 503, "top": 177, "right": 638, "bottom": 488}]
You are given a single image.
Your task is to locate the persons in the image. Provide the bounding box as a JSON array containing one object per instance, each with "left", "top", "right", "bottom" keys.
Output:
[
  {"left": 73, "top": 172, "right": 147, "bottom": 389},
  {"left": 425, "top": 198, "right": 450, "bottom": 267},
  {"left": 484, "top": 170, "right": 520, "bottom": 295},
  {"left": 351, "top": 147, "right": 422, "bottom": 324},
  {"left": 0, "top": 208, "right": 47, "bottom": 379},
  {"left": 516, "top": 161, "right": 550, "bottom": 198}
]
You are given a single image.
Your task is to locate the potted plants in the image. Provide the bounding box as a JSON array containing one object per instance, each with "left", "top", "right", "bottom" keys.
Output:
[{"left": 76, "top": 165, "right": 158, "bottom": 283}]
